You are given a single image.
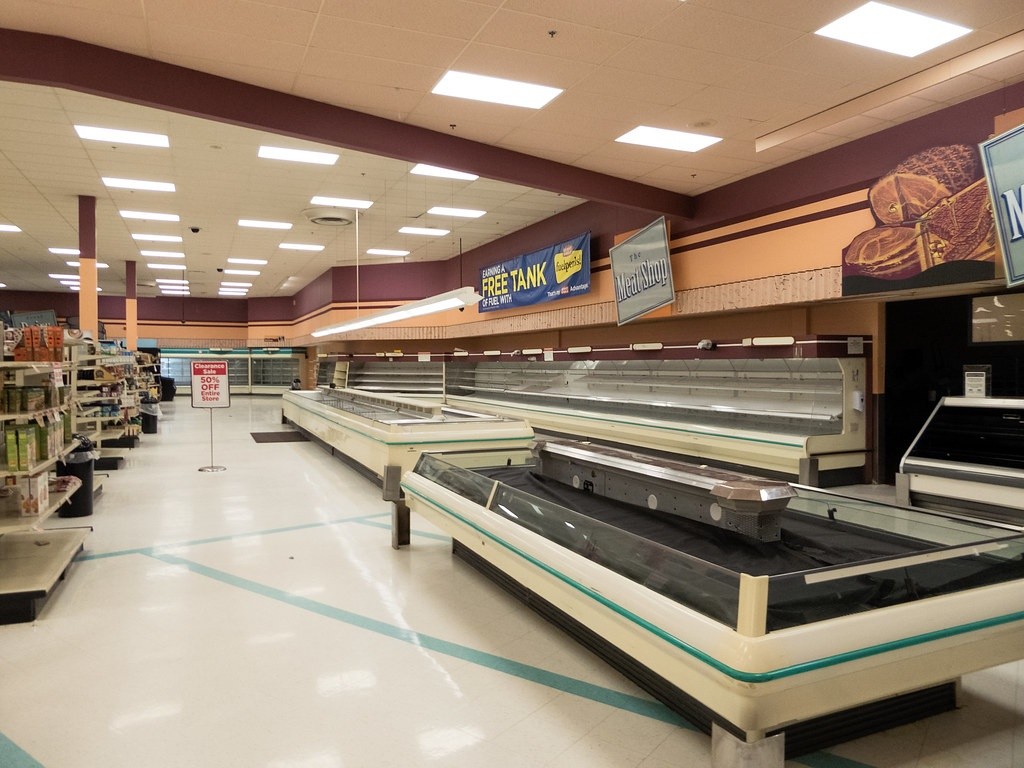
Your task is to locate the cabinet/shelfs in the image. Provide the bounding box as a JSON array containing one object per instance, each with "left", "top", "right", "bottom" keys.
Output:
[
  {"left": 0, "top": 321, "right": 95, "bottom": 626},
  {"left": 71, "top": 337, "right": 131, "bottom": 470},
  {"left": 250, "top": 354, "right": 310, "bottom": 394},
  {"left": 132, "top": 361, "right": 162, "bottom": 403},
  {"left": 162, "top": 353, "right": 249, "bottom": 394},
  {"left": 63, "top": 337, "right": 113, "bottom": 501}
]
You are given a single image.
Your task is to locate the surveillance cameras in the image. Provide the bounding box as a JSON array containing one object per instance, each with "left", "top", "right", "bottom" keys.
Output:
[
  {"left": 190, "top": 228, "right": 199, "bottom": 233},
  {"left": 217, "top": 269, "right": 223, "bottom": 272}
]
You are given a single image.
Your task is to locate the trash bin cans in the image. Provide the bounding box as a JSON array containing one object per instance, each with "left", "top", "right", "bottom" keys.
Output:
[
  {"left": 160, "top": 377, "right": 177, "bottom": 401},
  {"left": 56, "top": 449, "right": 95, "bottom": 518},
  {"left": 140, "top": 397, "right": 159, "bottom": 434}
]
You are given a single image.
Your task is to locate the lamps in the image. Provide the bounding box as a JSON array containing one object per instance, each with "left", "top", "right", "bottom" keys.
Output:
[{"left": 310, "top": 286, "right": 484, "bottom": 338}]
